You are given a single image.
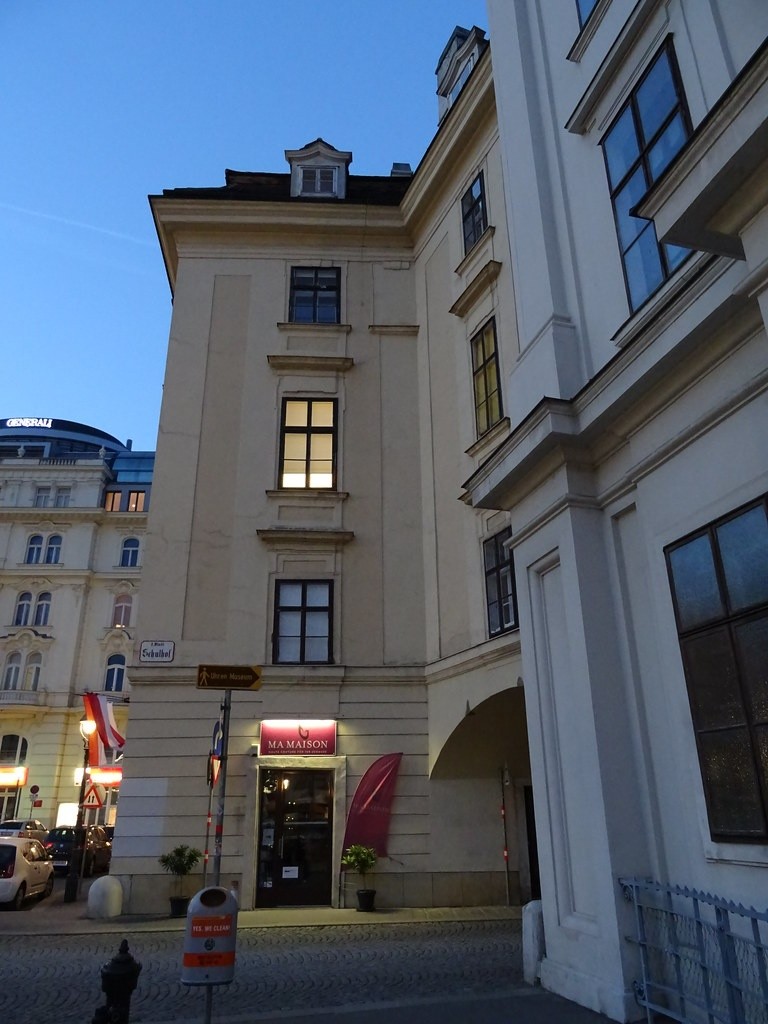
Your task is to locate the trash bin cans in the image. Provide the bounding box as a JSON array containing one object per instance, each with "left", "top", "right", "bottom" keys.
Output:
[{"left": 182, "top": 887, "right": 240, "bottom": 985}]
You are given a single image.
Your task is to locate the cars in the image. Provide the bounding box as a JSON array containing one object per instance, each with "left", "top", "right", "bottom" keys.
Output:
[
  {"left": 100, "top": 826, "right": 114, "bottom": 841},
  {"left": 0, "top": 817, "right": 50, "bottom": 844},
  {"left": 0, "top": 836, "right": 55, "bottom": 912},
  {"left": 40, "top": 825, "right": 113, "bottom": 877}
]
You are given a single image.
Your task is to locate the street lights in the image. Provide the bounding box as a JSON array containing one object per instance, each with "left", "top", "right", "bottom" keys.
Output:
[
  {"left": 10, "top": 764, "right": 25, "bottom": 820},
  {"left": 60, "top": 712, "right": 99, "bottom": 902}
]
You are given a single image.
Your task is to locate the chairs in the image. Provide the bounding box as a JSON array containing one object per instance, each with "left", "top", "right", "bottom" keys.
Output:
[{"left": 63, "top": 830, "right": 74, "bottom": 840}]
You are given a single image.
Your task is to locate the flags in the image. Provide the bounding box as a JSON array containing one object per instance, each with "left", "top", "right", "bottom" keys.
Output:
[
  {"left": 86, "top": 693, "right": 125, "bottom": 750},
  {"left": 213, "top": 709, "right": 222, "bottom": 788}
]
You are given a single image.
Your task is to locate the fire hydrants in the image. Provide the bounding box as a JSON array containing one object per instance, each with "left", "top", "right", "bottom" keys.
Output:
[{"left": 91, "top": 938, "right": 142, "bottom": 1024}]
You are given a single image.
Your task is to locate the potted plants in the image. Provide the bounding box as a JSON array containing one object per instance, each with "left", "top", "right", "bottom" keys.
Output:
[
  {"left": 341, "top": 844, "right": 378, "bottom": 908},
  {"left": 159, "top": 844, "right": 201, "bottom": 915}
]
